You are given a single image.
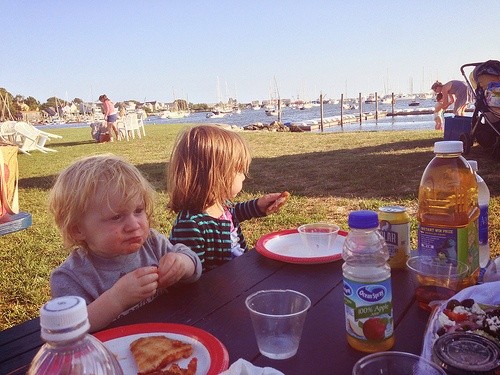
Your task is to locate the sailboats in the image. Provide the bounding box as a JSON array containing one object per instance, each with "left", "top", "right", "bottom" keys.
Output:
[
  {"left": 251, "top": 78, "right": 321, "bottom": 116},
  {"left": 42, "top": 83, "right": 105, "bottom": 126},
  {"left": 205, "top": 75, "right": 241, "bottom": 118},
  {"left": 157, "top": 87, "right": 191, "bottom": 119},
  {"left": 328, "top": 63, "right": 438, "bottom": 110}
]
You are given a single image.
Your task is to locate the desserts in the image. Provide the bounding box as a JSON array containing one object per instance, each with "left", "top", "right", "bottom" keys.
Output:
[{"left": 436, "top": 298, "right": 500, "bottom": 345}]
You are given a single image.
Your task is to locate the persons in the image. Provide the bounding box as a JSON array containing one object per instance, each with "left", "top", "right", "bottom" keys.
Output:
[
  {"left": 165, "top": 125, "right": 290, "bottom": 274},
  {"left": 99, "top": 94, "right": 120, "bottom": 143},
  {"left": 48, "top": 155, "right": 202, "bottom": 333},
  {"left": 431, "top": 79, "right": 474, "bottom": 130}
]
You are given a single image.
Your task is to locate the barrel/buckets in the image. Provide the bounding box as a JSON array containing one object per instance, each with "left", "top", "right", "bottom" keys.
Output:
[{"left": 444, "top": 116, "right": 473, "bottom": 155}]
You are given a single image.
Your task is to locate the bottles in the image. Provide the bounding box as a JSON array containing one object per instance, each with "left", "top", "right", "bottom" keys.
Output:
[
  {"left": 468, "top": 160, "right": 490, "bottom": 270},
  {"left": 417, "top": 140, "right": 479, "bottom": 313},
  {"left": 342, "top": 211, "right": 394, "bottom": 353},
  {"left": 26, "top": 296, "right": 124, "bottom": 375}
]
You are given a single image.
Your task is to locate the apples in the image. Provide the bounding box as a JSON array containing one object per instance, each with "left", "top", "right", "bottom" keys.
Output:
[{"left": 349, "top": 318, "right": 386, "bottom": 340}]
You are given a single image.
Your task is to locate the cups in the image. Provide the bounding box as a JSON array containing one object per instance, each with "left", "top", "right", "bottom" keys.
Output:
[
  {"left": 297, "top": 223, "right": 340, "bottom": 256},
  {"left": 406, "top": 255, "right": 469, "bottom": 313},
  {"left": 352, "top": 351, "right": 447, "bottom": 375},
  {"left": 432, "top": 332, "right": 500, "bottom": 375},
  {"left": 245, "top": 289, "right": 312, "bottom": 360}
]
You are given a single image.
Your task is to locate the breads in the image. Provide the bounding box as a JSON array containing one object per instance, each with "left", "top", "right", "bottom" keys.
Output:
[{"left": 128, "top": 335, "right": 193, "bottom": 373}]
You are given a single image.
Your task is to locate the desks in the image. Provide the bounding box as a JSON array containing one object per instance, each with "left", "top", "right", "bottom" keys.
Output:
[{"left": 11, "top": 245, "right": 432, "bottom": 375}]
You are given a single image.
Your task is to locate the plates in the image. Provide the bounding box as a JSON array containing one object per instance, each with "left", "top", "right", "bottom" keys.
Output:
[
  {"left": 255, "top": 230, "right": 365, "bottom": 264},
  {"left": 38, "top": 323, "right": 229, "bottom": 375}
]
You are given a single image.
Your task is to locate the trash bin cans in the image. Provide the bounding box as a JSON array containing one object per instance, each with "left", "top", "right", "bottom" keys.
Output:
[{"left": 443, "top": 115, "right": 474, "bottom": 149}]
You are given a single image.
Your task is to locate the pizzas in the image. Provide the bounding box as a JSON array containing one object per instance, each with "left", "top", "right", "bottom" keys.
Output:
[{"left": 266, "top": 191, "right": 290, "bottom": 215}]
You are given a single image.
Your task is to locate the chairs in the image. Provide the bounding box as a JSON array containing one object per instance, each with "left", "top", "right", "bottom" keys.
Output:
[
  {"left": 0, "top": 121, "right": 62, "bottom": 155},
  {"left": 116, "top": 113, "right": 146, "bottom": 141}
]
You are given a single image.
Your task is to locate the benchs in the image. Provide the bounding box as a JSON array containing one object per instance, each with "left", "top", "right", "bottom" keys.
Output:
[{"left": 0, "top": 316, "right": 46, "bottom": 375}]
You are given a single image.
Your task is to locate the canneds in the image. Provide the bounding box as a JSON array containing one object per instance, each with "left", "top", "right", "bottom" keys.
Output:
[{"left": 376, "top": 206, "right": 410, "bottom": 270}]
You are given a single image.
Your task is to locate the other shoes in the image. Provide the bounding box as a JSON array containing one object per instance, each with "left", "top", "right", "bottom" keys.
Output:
[
  {"left": 108, "top": 136, "right": 113, "bottom": 142},
  {"left": 116, "top": 135, "right": 120, "bottom": 140}
]
